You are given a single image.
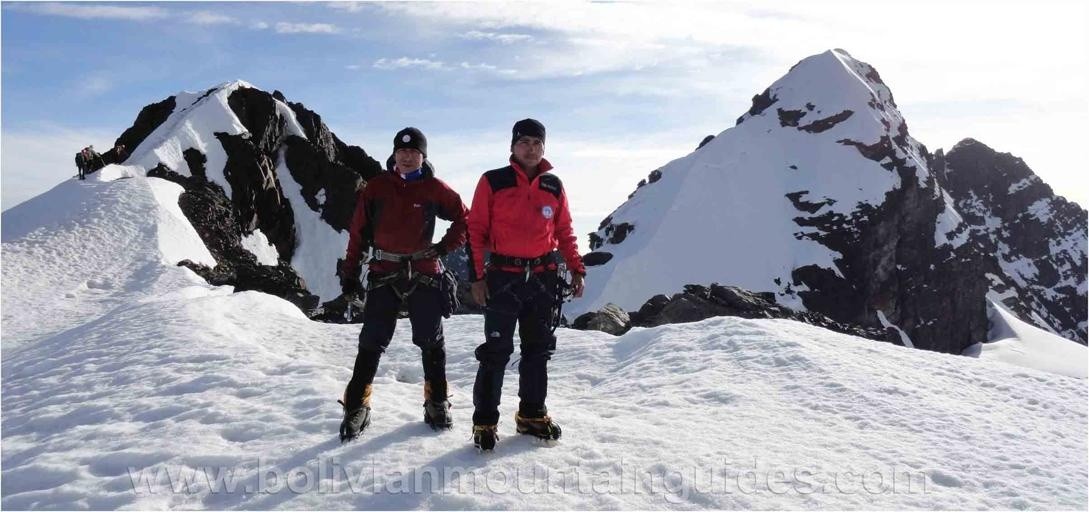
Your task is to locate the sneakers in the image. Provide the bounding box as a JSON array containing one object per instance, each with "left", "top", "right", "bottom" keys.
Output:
[
  {"left": 338, "top": 399, "right": 372, "bottom": 438},
  {"left": 515, "top": 405, "right": 562, "bottom": 442},
  {"left": 424, "top": 394, "right": 454, "bottom": 428},
  {"left": 472, "top": 420, "right": 499, "bottom": 451}
]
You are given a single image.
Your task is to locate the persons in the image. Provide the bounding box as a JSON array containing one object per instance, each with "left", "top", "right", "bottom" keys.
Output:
[
  {"left": 464, "top": 118, "right": 586, "bottom": 451},
  {"left": 339, "top": 128, "right": 470, "bottom": 439}
]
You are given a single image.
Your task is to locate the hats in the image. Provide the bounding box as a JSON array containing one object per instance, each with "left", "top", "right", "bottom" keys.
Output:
[
  {"left": 510, "top": 117, "right": 546, "bottom": 148},
  {"left": 392, "top": 126, "right": 428, "bottom": 160}
]
